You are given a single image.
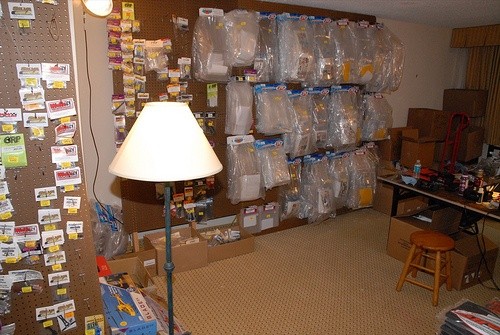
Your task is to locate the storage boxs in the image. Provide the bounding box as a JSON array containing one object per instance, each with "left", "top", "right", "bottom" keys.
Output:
[
  {"left": 386, "top": 204, "right": 462, "bottom": 272},
  {"left": 405, "top": 106, "right": 452, "bottom": 142},
  {"left": 450, "top": 125, "right": 485, "bottom": 163},
  {"left": 98, "top": 272, "right": 158, "bottom": 335},
  {"left": 398, "top": 139, "right": 436, "bottom": 171},
  {"left": 424, "top": 228, "right": 499, "bottom": 291},
  {"left": 93, "top": 254, "right": 159, "bottom": 300},
  {"left": 435, "top": 141, "right": 454, "bottom": 162},
  {"left": 442, "top": 88, "right": 489, "bottom": 119},
  {"left": 374, "top": 163, "right": 430, "bottom": 217},
  {"left": 373, "top": 126, "right": 420, "bottom": 162},
  {"left": 196, "top": 222, "right": 256, "bottom": 264},
  {"left": 95, "top": 237, "right": 158, "bottom": 283},
  {"left": 144, "top": 225, "right": 209, "bottom": 276}
]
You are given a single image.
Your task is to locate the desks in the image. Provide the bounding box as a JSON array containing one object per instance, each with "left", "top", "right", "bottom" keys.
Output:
[{"left": 376, "top": 166, "right": 500, "bottom": 257}]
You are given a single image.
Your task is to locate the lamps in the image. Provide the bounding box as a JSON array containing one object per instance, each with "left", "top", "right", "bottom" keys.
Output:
[
  {"left": 106, "top": 100, "right": 227, "bottom": 335},
  {"left": 74, "top": 0, "right": 125, "bottom": 227}
]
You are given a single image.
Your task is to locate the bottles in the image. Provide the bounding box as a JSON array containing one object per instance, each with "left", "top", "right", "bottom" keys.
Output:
[
  {"left": 413, "top": 159, "right": 422, "bottom": 181},
  {"left": 473, "top": 168, "right": 483, "bottom": 190},
  {"left": 476, "top": 188, "right": 485, "bottom": 204}
]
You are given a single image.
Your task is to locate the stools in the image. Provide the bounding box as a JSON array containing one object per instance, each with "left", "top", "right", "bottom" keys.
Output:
[{"left": 396, "top": 230, "right": 456, "bottom": 307}]
